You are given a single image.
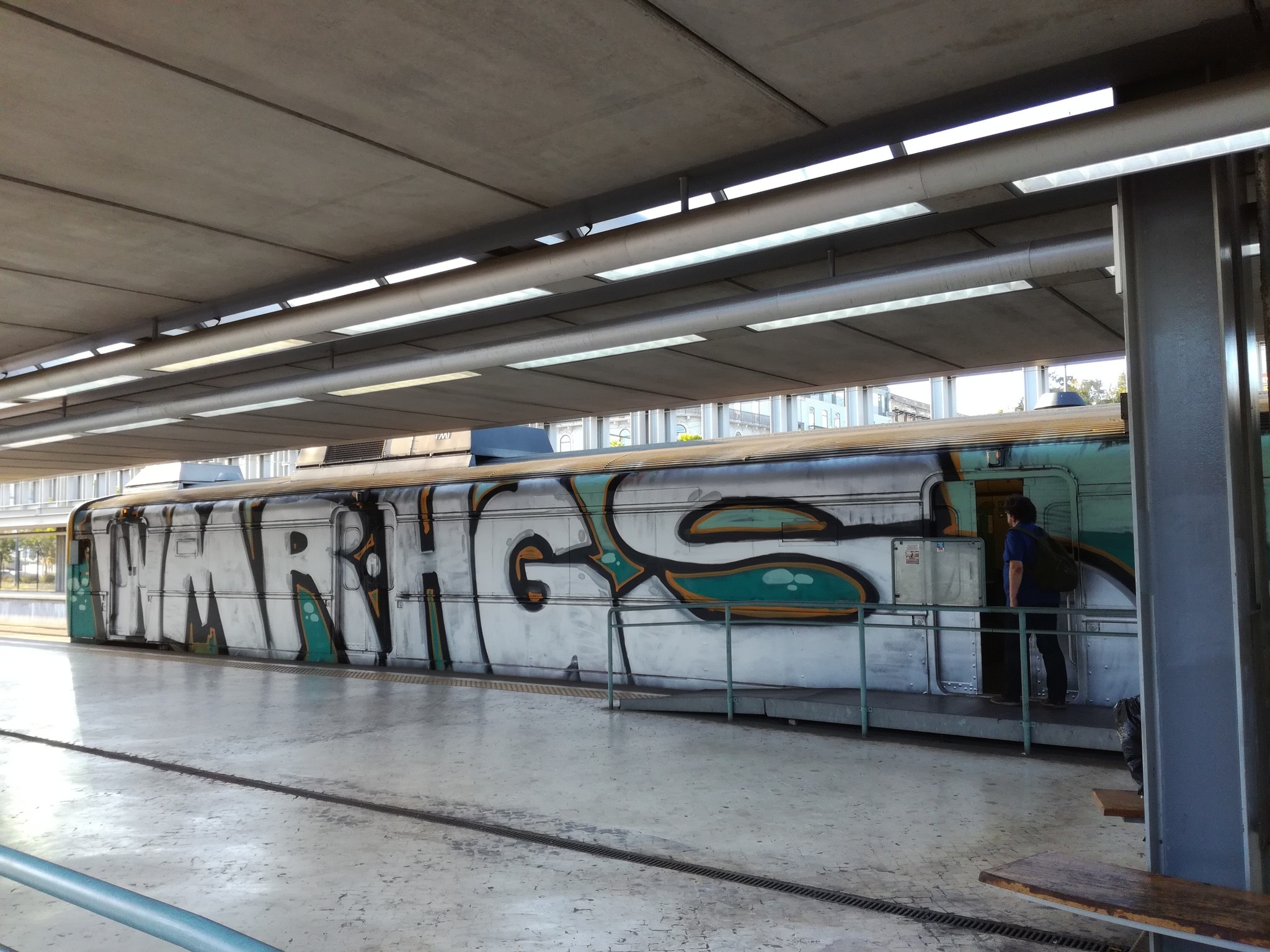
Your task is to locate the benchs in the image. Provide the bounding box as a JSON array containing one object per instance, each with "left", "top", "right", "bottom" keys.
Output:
[
  {"left": 977, "top": 850, "right": 1270, "bottom": 952},
  {"left": 1092, "top": 789, "right": 1145, "bottom": 823}
]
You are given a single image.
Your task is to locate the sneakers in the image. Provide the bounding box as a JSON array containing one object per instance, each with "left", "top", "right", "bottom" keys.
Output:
[
  {"left": 1041, "top": 695, "right": 1066, "bottom": 709},
  {"left": 990, "top": 694, "right": 1021, "bottom": 706}
]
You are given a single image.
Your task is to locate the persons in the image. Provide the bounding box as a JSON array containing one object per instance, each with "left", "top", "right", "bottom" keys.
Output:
[
  {"left": 991, "top": 496, "right": 1068, "bottom": 709},
  {"left": 1112, "top": 695, "right": 1144, "bottom": 797}
]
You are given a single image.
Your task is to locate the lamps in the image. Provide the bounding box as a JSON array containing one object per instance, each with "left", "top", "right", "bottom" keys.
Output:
[{"left": 0, "top": 73, "right": 1270, "bottom": 449}]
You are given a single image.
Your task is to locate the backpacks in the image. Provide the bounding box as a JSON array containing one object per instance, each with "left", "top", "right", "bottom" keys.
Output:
[{"left": 1005, "top": 526, "right": 1079, "bottom": 592}]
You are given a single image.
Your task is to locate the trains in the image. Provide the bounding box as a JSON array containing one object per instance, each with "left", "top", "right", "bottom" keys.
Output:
[{"left": 64, "top": 386, "right": 1268, "bottom": 702}]
